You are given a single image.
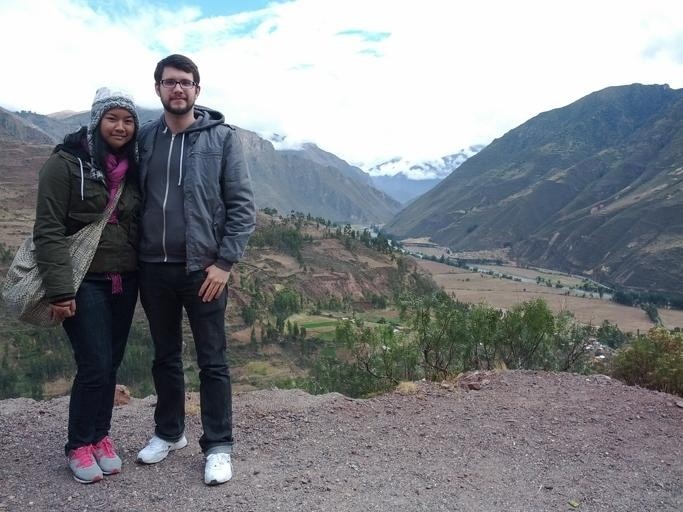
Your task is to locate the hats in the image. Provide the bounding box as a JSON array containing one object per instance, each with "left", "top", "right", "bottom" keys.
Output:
[{"left": 88, "top": 86, "right": 140, "bottom": 136}]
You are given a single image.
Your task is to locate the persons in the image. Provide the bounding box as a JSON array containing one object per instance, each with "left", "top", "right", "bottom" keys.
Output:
[
  {"left": 31, "top": 86, "right": 145, "bottom": 487},
  {"left": 126, "top": 54, "right": 257, "bottom": 486}
]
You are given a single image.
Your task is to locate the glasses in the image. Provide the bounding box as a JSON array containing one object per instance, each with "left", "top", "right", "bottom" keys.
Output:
[{"left": 160, "top": 78, "right": 197, "bottom": 89}]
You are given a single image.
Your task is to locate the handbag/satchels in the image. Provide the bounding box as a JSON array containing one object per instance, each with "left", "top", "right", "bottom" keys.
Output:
[{"left": 2, "top": 224, "right": 102, "bottom": 328}]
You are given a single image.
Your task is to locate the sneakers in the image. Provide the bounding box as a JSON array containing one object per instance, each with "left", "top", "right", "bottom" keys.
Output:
[
  {"left": 68, "top": 444, "right": 104, "bottom": 484},
  {"left": 92, "top": 435, "right": 122, "bottom": 475},
  {"left": 137, "top": 431, "right": 188, "bottom": 464},
  {"left": 204, "top": 446, "right": 232, "bottom": 485}
]
